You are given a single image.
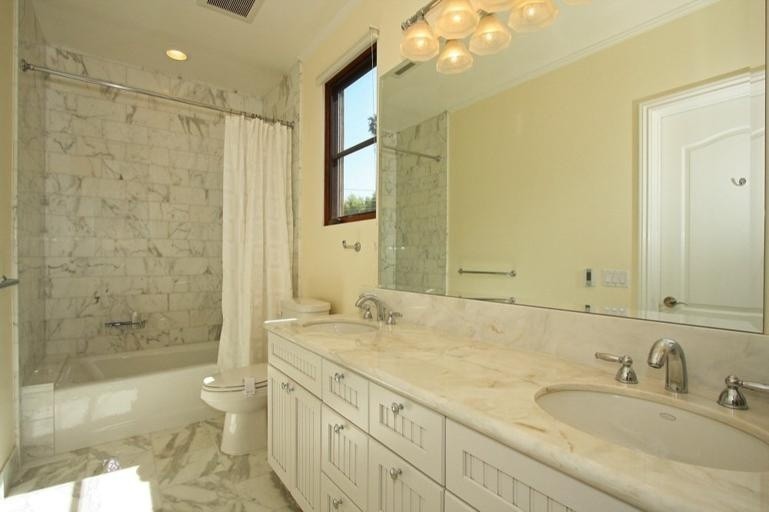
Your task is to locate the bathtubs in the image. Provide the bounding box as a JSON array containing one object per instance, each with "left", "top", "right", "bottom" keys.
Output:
[{"left": 55, "top": 339, "right": 221, "bottom": 456}]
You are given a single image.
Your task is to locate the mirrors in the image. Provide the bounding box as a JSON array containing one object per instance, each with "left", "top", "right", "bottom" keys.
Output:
[{"left": 378, "top": 1, "right": 768, "bottom": 335}]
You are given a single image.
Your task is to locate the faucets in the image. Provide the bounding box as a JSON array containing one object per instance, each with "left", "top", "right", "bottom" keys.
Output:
[
  {"left": 354, "top": 295, "right": 385, "bottom": 321},
  {"left": 648, "top": 337, "right": 687, "bottom": 393}
]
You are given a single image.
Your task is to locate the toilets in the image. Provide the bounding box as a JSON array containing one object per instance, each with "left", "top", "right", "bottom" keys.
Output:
[{"left": 201, "top": 297, "right": 331, "bottom": 456}]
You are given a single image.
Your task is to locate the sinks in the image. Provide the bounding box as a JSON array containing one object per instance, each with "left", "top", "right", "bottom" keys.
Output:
[
  {"left": 302, "top": 320, "right": 380, "bottom": 337},
  {"left": 534, "top": 383, "right": 769, "bottom": 475}
]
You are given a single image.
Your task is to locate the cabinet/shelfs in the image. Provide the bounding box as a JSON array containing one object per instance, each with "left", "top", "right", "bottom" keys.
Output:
[
  {"left": 321, "top": 358, "right": 368, "bottom": 512},
  {"left": 367, "top": 381, "right": 444, "bottom": 511},
  {"left": 444, "top": 418, "right": 647, "bottom": 512},
  {"left": 267, "top": 331, "right": 321, "bottom": 512}
]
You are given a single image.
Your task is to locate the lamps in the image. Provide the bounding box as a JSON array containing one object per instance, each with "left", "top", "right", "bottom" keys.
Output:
[
  {"left": 392, "top": 0, "right": 559, "bottom": 65},
  {"left": 435, "top": 1, "right": 559, "bottom": 77}
]
